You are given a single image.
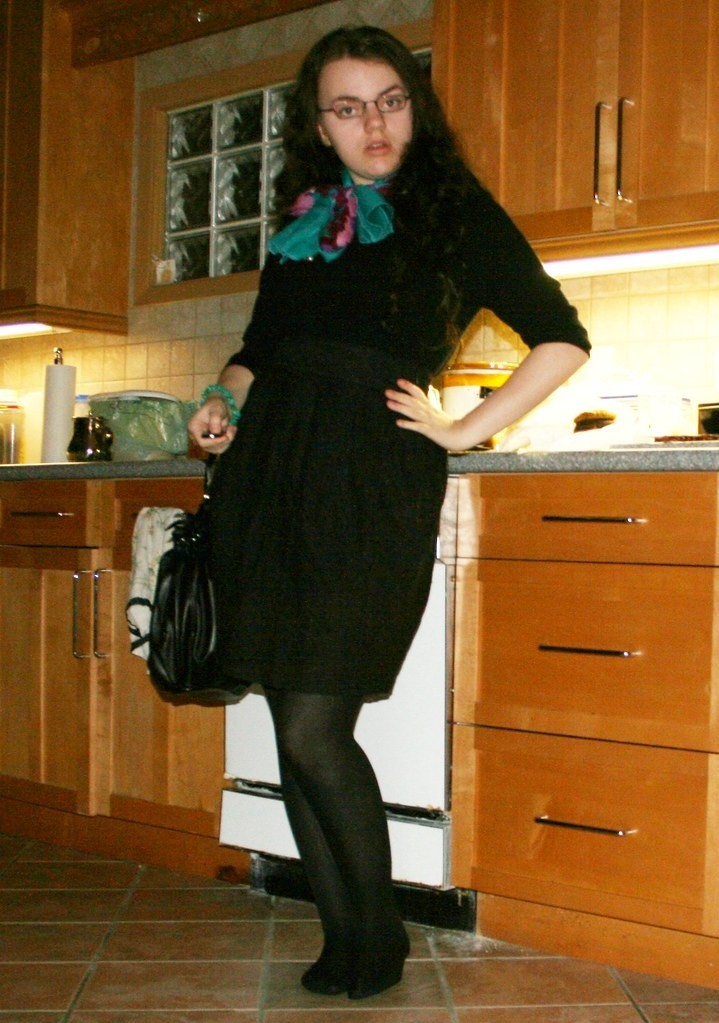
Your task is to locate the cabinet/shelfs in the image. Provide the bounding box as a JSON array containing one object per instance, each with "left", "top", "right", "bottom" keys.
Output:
[
  {"left": 0, "top": 477, "right": 246, "bottom": 881},
  {"left": 452, "top": 470, "right": 719, "bottom": 990},
  {"left": 0, "top": 0, "right": 136, "bottom": 338},
  {"left": 430, "top": 0, "right": 719, "bottom": 263}
]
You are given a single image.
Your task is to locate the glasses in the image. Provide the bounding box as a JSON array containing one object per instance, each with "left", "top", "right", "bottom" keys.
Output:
[{"left": 317, "top": 93, "right": 411, "bottom": 120}]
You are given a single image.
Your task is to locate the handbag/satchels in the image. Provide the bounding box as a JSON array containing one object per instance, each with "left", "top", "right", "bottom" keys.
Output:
[{"left": 125, "top": 448, "right": 252, "bottom": 708}]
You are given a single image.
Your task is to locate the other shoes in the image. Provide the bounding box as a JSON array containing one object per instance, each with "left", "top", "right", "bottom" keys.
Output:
[
  {"left": 347, "top": 927, "right": 411, "bottom": 1000},
  {"left": 301, "top": 922, "right": 364, "bottom": 997}
]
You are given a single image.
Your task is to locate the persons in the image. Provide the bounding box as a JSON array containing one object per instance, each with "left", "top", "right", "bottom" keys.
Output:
[{"left": 184, "top": 24, "right": 592, "bottom": 1001}]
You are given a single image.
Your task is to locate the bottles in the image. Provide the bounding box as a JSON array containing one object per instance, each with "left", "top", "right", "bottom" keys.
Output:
[
  {"left": 66, "top": 417, "right": 90, "bottom": 462},
  {"left": 0, "top": 401, "right": 27, "bottom": 464}
]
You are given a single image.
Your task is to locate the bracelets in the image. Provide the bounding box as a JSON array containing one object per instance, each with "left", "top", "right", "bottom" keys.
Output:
[{"left": 198, "top": 383, "right": 239, "bottom": 428}]
